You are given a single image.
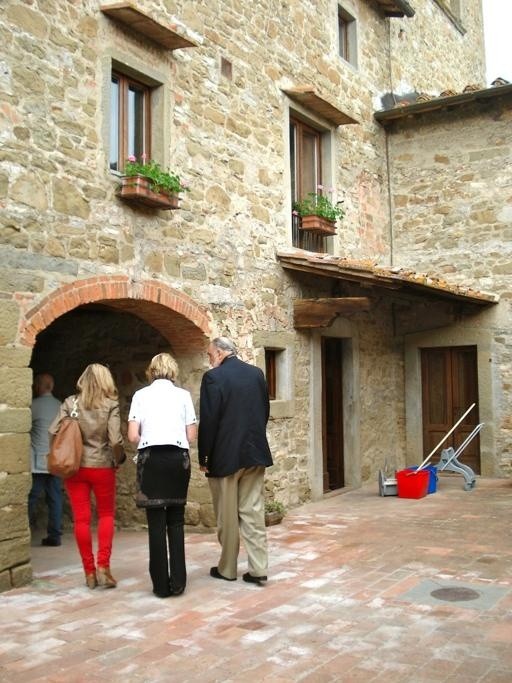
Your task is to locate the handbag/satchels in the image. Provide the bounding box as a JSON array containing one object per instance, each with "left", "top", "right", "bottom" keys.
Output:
[{"left": 46, "top": 395, "right": 84, "bottom": 478}]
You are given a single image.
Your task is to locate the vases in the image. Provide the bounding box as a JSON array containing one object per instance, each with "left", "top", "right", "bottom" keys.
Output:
[
  {"left": 120, "top": 175, "right": 179, "bottom": 210},
  {"left": 300, "top": 214, "right": 336, "bottom": 236}
]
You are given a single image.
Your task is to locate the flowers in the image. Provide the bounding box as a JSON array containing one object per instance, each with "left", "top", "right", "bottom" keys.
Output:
[
  {"left": 292, "top": 185, "right": 347, "bottom": 222},
  {"left": 122, "top": 153, "right": 192, "bottom": 195}
]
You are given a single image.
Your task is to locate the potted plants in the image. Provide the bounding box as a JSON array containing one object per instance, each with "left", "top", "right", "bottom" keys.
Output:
[{"left": 263, "top": 499, "right": 287, "bottom": 527}]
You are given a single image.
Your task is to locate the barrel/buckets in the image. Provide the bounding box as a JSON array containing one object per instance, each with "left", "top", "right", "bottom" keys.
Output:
[
  {"left": 395, "top": 470, "right": 430, "bottom": 499},
  {"left": 413, "top": 464, "right": 438, "bottom": 495}
]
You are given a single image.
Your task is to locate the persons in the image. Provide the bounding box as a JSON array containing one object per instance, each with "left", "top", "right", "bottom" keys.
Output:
[
  {"left": 196, "top": 336, "right": 273, "bottom": 584},
  {"left": 49, "top": 364, "right": 126, "bottom": 589},
  {"left": 29, "top": 373, "right": 63, "bottom": 546},
  {"left": 128, "top": 353, "right": 197, "bottom": 599}
]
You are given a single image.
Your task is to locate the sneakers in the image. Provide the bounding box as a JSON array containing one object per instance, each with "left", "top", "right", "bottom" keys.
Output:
[
  {"left": 243, "top": 572, "right": 269, "bottom": 586},
  {"left": 209, "top": 566, "right": 238, "bottom": 582},
  {"left": 40, "top": 535, "right": 62, "bottom": 548}
]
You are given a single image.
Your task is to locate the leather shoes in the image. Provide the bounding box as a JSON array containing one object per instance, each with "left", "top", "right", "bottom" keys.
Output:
[
  {"left": 85, "top": 573, "right": 98, "bottom": 590},
  {"left": 97, "top": 566, "right": 117, "bottom": 590}
]
